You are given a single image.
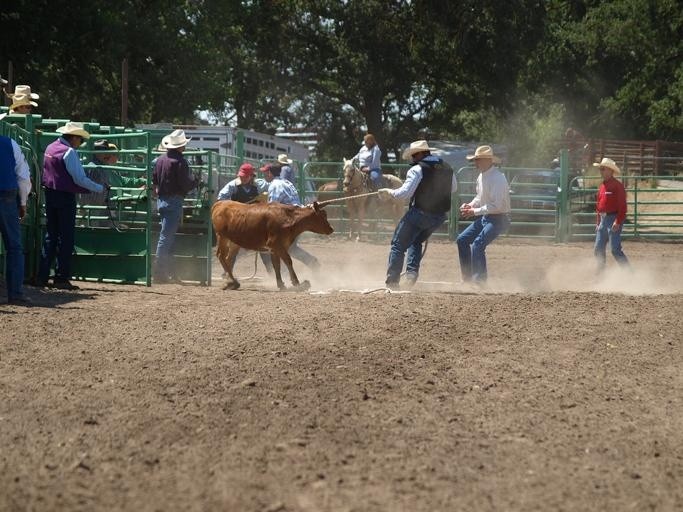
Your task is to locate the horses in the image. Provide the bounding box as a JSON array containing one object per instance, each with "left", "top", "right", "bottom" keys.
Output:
[{"left": 317, "top": 156, "right": 408, "bottom": 242}]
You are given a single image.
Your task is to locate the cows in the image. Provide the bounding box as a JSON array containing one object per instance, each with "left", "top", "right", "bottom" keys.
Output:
[{"left": 210, "top": 199, "right": 334, "bottom": 292}]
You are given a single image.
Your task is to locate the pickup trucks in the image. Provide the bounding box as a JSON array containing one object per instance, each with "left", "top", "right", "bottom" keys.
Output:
[{"left": 507, "top": 170, "right": 597, "bottom": 224}]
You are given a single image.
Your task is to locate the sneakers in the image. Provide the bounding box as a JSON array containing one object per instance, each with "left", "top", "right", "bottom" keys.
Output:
[{"left": 54, "top": 281, "right": 80, "bottom": 290}]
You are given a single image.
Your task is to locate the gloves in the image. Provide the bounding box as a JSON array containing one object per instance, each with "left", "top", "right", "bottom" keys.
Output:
[{"left": 379, "top": 188, "right": 392, "bottom": 201}]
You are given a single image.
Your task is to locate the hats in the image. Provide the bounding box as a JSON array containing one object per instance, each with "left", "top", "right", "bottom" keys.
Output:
[
  {"left": 593, "top": 157, "right": 620, "bottom": 175},
  {"left": 402, "top": 140, "right": 436, "bottom": 159},
  {"left": 56, "top": 122, "right": 90, "bottom": 139},
  {"left": 9, "top": 94, "right": 38, "bottom": 110},
  {"left": 362, "top": 135, "right": 375, "bottom": 145},
  {"left": 238, "top": 154, "right": 293, "bottom": 176},
  {"left": 162, "top": 129, "right": 192, "bottom": 149},
  {"left": 94, "top": 140, "right": 115, "bottom": 150},
  {"left": 7, "top": 84, "right": 40, "bottom": 100},
  {"left": 466, "top": 146, "right": 499, "bottom": 163}
]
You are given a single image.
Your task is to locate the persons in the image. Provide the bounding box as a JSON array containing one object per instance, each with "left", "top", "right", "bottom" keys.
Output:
[
  {"left": 377, "top": 140, "right": 458, "bottom": 289},
  {"left": 78, "top": 138, "right": 147, "bottom": 228},
  {"left": 36, "top": 123, "right": 104, "bottom": 289},
  {"left": 351, "top": 133, "right": 382, "bottom": 192},
  {"left": 0, "top": 135, "right": 32, "bottom": 305},
  {"left": 455, "top": 145, "right": 511, "bottom": 282},
  {"left": 152, "top": 129, "right": 201, "bottom": 284},
  {"left": 9, "top": 86, "right": 40, "bottom": 115},
  {"left": 591, "top": 157, "right": 629, "bottom": 275},
  {"left": 217, "top": 154, "right": 322, "bottom": 278}
]
